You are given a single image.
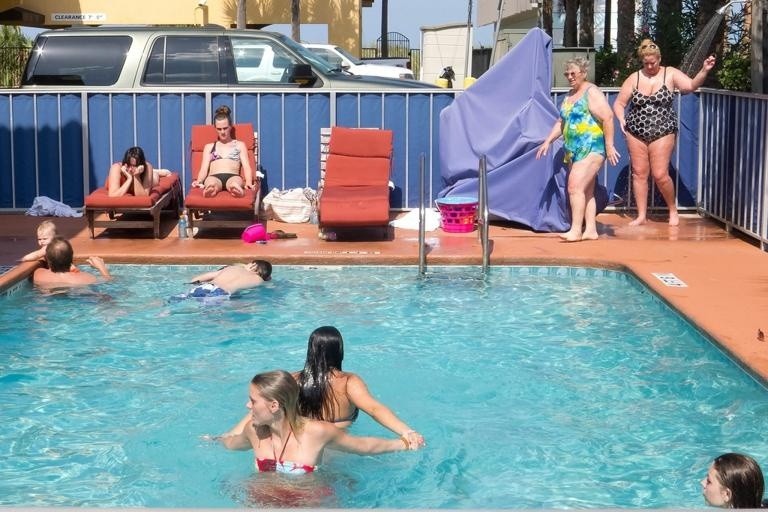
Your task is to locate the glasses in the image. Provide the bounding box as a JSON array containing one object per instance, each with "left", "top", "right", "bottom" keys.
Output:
[
  {"left": 641, "top": 45, "right": 657, "bottom": 50},
  {"left": 564, "top": 72, "right": 580, "bottom": 78}
]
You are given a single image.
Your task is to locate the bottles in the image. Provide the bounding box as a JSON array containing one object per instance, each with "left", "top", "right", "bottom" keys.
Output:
[
  {"left": 311, "top": 205, "right": 319, "bottom": 225},
  {"left": 178, "top": 215, "right": 187, "bottom": 238}
]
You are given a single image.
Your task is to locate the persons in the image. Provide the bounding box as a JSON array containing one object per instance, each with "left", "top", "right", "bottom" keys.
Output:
[
  {"left": 190, "top": 107, "right": 257, "bottom": 198},
  {"left": 111, "top": 260, "right": 273, "bottom": 319},
  {"left": 19, "top": 220, "right": 79, "bottom": 276},
  {"left": 534, "top": 55, "right": 622, "bottom": 242},
  {"left": 289, "top": 325, "right": 424, "bottom": 448},
  {"left": 107, "top": 147, "right": 173, "bottom": 198},
  {"left": 31, "top": 238, "right": 116, "bottom": 300},
  {"left": 698, "top": 454, "right": 768, "bottom": 507},
  {"left": 611, "top": 38, "right": 717, "bottom": 227},
  {"left": 209, "top": 370, "right": 418, "bottom": 503}
]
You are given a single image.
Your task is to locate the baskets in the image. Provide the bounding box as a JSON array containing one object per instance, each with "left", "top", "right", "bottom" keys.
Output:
[{"left": 434, "top": 198, "right": 478, "bottom": 234}]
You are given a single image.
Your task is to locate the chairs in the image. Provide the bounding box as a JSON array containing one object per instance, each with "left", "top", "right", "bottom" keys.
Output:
[
  {"left": 83, "top": 166, "right": 182, "bottom": 242},
  {"left": 316, "top": 125, "right": 393, "bottom": 234},
  {"left": 184, "top": 122, "right": 264, "bottom": 238}
]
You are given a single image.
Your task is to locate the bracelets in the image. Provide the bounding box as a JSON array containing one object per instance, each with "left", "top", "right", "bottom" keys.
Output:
[{"left": 400, "top": 437, "right": 409, "bottom": 451}]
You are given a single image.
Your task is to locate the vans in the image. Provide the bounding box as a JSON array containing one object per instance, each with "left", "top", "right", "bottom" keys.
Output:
[
  {"left": 229, "top": 44, "right": 416, "bottom": 82},
  {"left": 18, "top": 28, "right": 455, "bottom": 97}
]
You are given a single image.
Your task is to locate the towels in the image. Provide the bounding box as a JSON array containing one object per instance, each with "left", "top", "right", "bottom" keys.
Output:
[
  {"left": 23, "top": 195, "right": 84, "bottom": 219},
  {"left": 389, "top": 207, "right": 441, "bottom": 234}
]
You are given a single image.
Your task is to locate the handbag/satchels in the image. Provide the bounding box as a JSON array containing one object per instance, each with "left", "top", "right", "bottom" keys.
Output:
[{"left": 260, "top": 187, "right": 316, "bottom": 224}]
